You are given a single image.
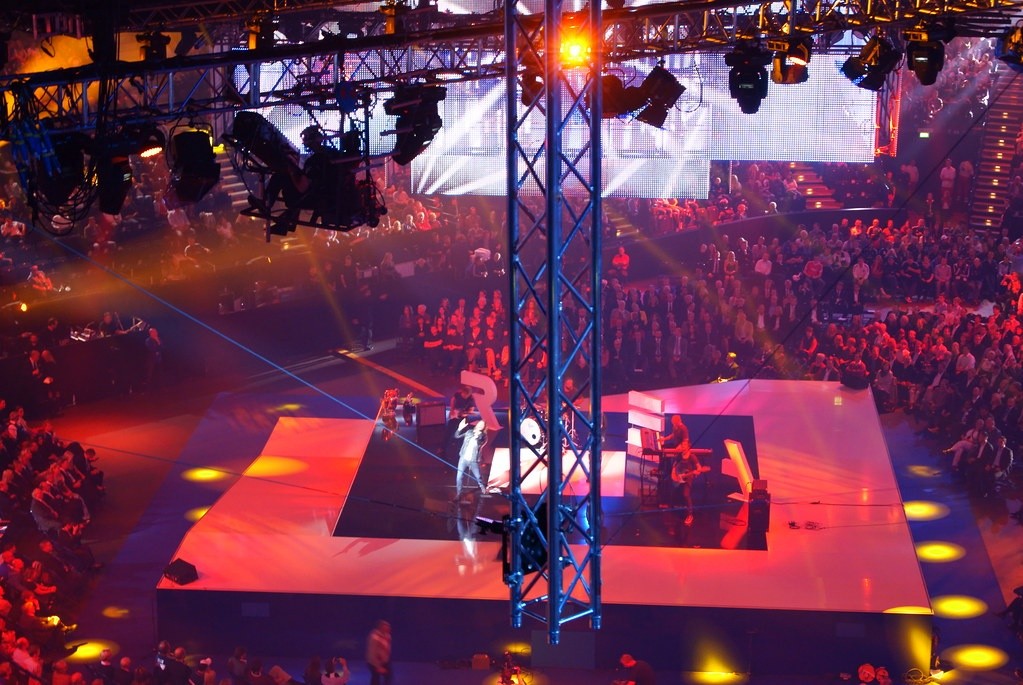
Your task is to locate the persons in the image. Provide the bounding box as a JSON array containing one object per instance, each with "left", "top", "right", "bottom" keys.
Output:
[
  {"left": 672, "top": 438, "right": 699, "bottom": 524},
  {"left": 658, "top": 414, "right": 688, "bottom": 481},
  {"left": 452, "top": 416, "right": 490, "bottom": 498},
  {"left": 439, "top": 383, "right": 475, "bottom": 454},
  {"left": 584, "top": 406, "right": 608, "bottom": 483},
  {"left": 0, "top": 175, "right": 1021, "bottom": 394},
  {"left": 555, "top": 376, "right": 582, "bottom": 425},
  {"left": 2, "top": 400, "right": 408, "bottom": 685},
  {"left": 789, "top": 289, "right": 1022, "bottom": 514}
]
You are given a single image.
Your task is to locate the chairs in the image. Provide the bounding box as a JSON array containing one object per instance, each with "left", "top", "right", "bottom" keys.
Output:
[{"left": 1, "top": 159, "right": 845, "bottom": 341}]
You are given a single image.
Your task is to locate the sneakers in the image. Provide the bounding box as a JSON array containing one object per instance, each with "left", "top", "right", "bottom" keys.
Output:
[
  {"left": 941, "top": 447, "right": 953, "bottom": 456},
  {"left": 453, "top": 490, "right": 465, "bottom": 503},
  {"left": 436, "top": 446, "right": 446, "bottom": 455},
  {"left": 658, "top": 502, "right": 671, "bottom": 509},
  {"left": 683, "top": 513, "right": 696, "bottom": 525},
  {"left": 908, "top": 402, "right": 916, "bottom": 412},
  {"left": 928, "top": 424, "right": 941, "bottom": 435}
]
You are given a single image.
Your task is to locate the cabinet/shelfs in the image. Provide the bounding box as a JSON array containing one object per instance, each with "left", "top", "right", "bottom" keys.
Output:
[{"left": 416, "top": 400, "right": 447, "bottom": 426}]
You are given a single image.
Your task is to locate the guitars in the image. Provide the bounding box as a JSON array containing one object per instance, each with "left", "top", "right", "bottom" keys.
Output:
[
  {"left": 671, "top": 467, "right": 710, "bottom": 486},
  {"left": 449, "top": 409, "right": 476, "bottom": 419}
]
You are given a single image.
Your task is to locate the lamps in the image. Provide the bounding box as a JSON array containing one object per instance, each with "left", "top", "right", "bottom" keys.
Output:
[
  {"left": 995, "top": 26, "right": 1023, "bottom": 65},
  {"left": 139, "top": 140, "right": 163, "bottom": 158},
  {"left": 722, "top": 42, "right": 773, "bottom": 114},
  {"left": 906, "top": 39, "right": 946, "bottom": 86},
  {"left": 584, "top": 74, "right": 624, "bottom": 118},
  {"left": 625, "top": 65, "right": 687, "bottom": 129},
  {"left": 840, "top": 35, "right": 902, "bottom": 91},
  {"left": 163, "top": 123, "right": 221, "bottom": 211},
  {"left": 788, "top": 35, "right": 815, "bottom": 66}
]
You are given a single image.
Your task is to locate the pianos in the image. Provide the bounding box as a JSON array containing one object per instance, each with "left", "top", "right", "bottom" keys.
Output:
[{"left": 662, "top": 447, "right": 714, "bottom": 460}]
